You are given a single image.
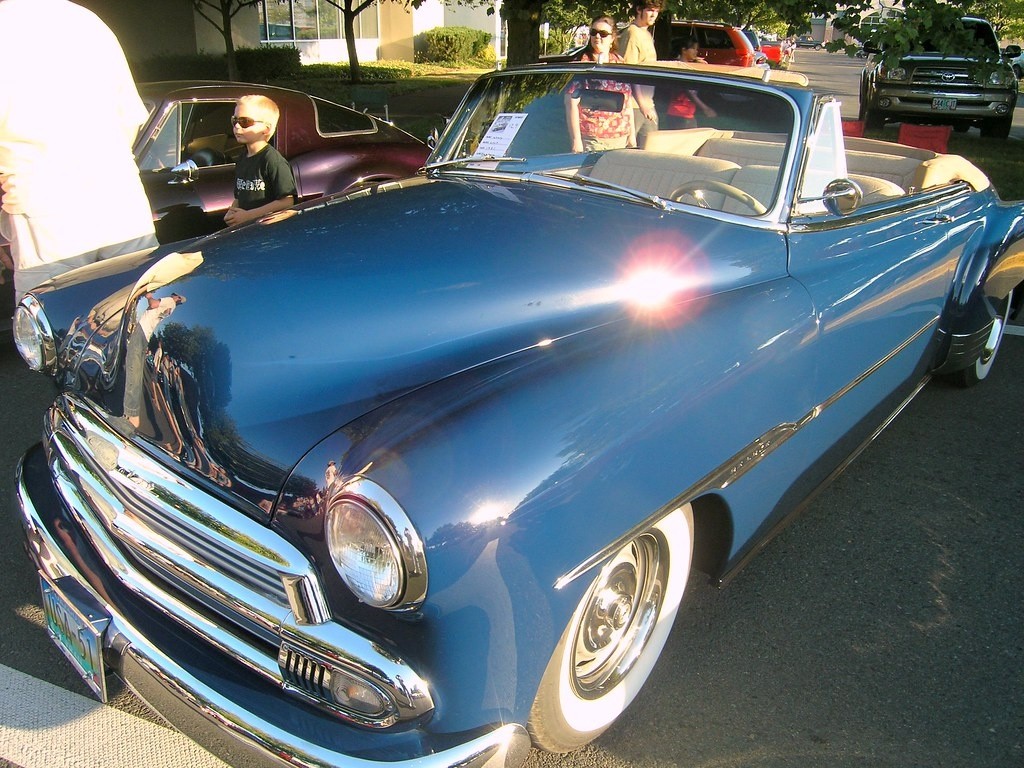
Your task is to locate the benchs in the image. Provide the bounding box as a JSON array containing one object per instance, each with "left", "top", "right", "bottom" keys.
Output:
[{"left": 588, "top": 138, "right": 924, "bottom": 216}]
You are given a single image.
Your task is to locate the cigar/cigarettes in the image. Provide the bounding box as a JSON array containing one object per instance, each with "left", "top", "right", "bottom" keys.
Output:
[{"left": 653, "top": 119, "right": 658, "bottom": 125}]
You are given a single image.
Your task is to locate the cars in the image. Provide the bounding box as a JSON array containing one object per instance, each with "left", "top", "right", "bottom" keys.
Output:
[
  {"left": 0, "top": 80, "right": 434, "bottom": 342},
  {"left": 795, "top": 35, "right": 822, "bottom": 51},
  {"left": 1001, "top": 47, "right": 1024, "bottom": 79},
  {"left": 858, "top": 17, "right": 1021, "bottom": 139},
  {"left": 11, "top": 62, "right": 1023, "bottom": 767},
  {"left": 539, "top": 19, "right": 796, "bottom": 70}
]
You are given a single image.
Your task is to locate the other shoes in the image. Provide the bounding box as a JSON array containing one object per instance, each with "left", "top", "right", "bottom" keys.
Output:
[{"left": 789, "top": 60, "right": 794, "bottom": 63}]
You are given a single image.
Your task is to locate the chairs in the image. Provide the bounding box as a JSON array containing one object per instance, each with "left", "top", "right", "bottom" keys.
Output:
[{"left": 190, "top": 147, "right": 221, "bottom": 168}]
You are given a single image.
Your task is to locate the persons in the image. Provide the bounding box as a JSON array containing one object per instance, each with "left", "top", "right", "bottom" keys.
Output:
[
  {"left": 615, "top": 0, "right": 664, "bottom": 147},
  {"left": 563, "top": 16, "right": 636, "bottom": 155},
  {"left": 223, "top": 95, "right": 296, "bottom": 229},
  {"left": 664, "top": 36, "right": 717, "bottom": 131},
  {"left": 783, "top": 38, "right": 796, "bottom": 64},
  {"left": 0, "top": 0, "right": 156, "bottom": 308}
]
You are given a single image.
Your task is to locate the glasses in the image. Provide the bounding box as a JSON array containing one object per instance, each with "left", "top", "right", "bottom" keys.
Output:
[
  {"left": 230, "top": 116, "right": 264, "bottom": 128},
  {"left": 589, "top": 28, "right": 613, "bottom": 38}
]
